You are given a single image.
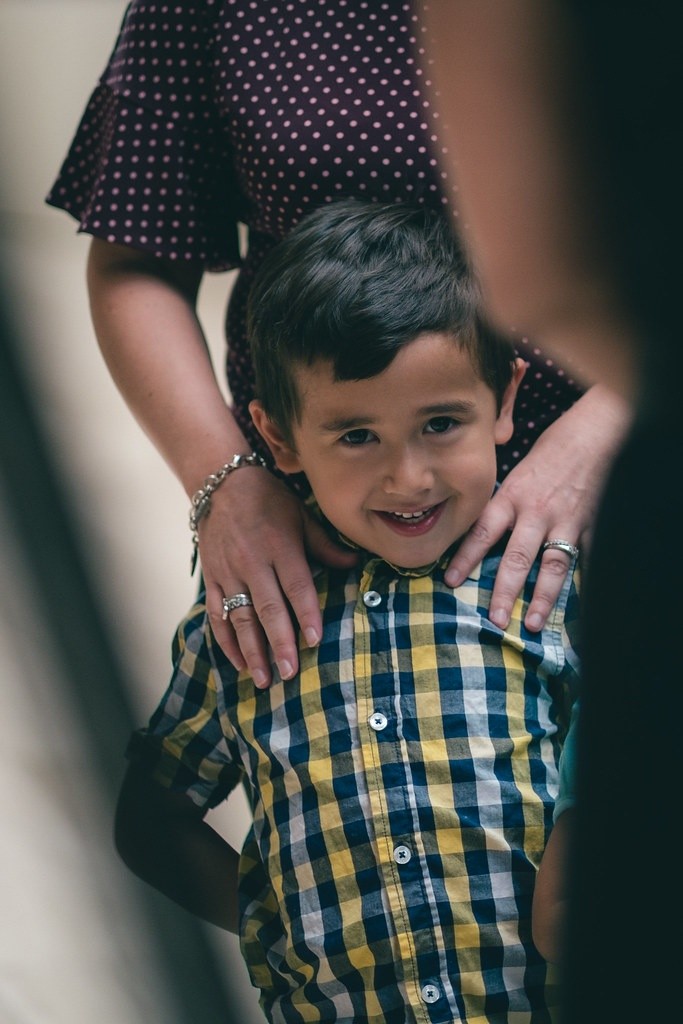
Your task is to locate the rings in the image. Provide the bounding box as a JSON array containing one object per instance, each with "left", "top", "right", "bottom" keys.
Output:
[
  {"left": 222, "top": 593, "right": 252, "bottom": 620},
  {"left": 543, "top": 539, "right": 579, "bottom": 561}
]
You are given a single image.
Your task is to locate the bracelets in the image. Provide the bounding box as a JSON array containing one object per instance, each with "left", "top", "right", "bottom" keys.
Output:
[{"left": 187, "top": 451, "right": 272, "bottom": 578}]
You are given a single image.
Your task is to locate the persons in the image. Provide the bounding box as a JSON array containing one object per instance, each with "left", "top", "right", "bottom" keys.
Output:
[
  {"left": 36, "top": 6, "right": 629, "bottom": 694},
  {"left": 112, "top": 207, "right": 571, "bottom": 1020},
  {"left": 425, "top": 0, "right": 674, "bottom": 1023}
]
